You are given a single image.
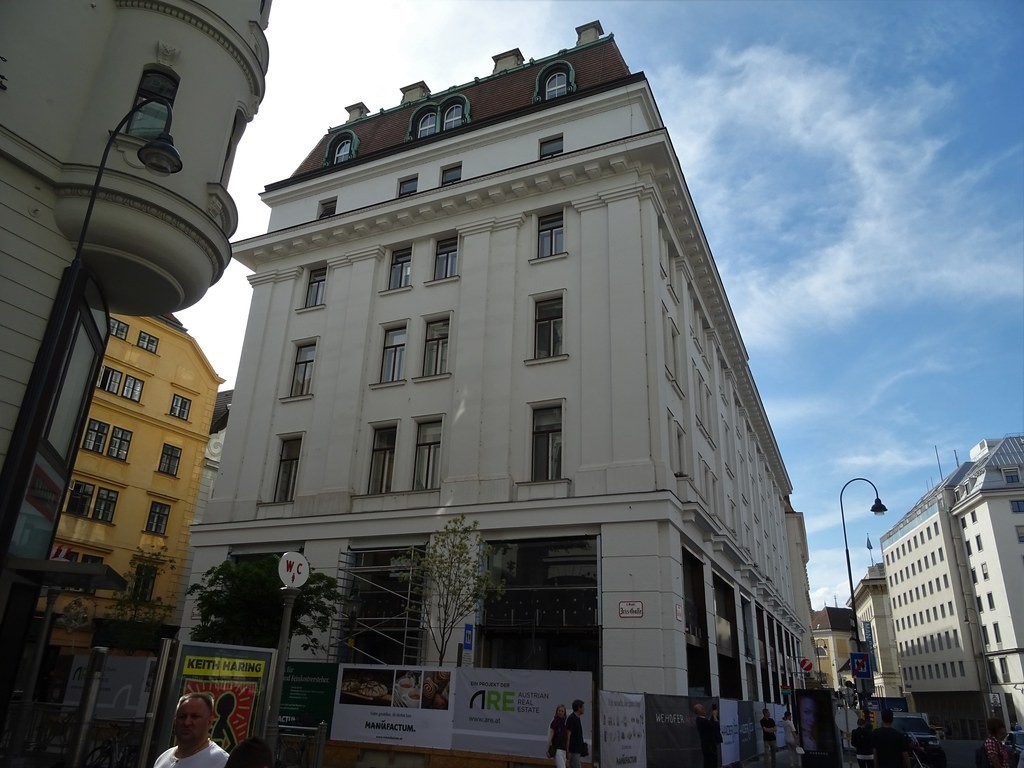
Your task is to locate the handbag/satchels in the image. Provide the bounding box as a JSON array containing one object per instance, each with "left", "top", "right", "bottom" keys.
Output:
[
  {"left": 548, "top": 745, "right": 556, "bottom": 757},
  {"left": 580, "top": 743, "right": 588, "bottom": 757},
  {"left": 796, "top": 745, "right": 805, "bottom": 755}
]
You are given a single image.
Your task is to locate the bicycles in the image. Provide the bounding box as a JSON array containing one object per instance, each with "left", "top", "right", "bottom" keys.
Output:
[{"left": 82, "top": 720, "right": 138, "bottom": 768}]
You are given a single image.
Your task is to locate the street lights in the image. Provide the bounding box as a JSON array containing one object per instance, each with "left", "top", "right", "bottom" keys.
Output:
[
  {"left": 963, "top": 608, "right": 996, "bottom": 722},
  {"left": 1, "top": 93, "right": 184, "bottom": 567},
  {"left": 841, "top": 477, "right": 887, "bottom": 728},
  {"left": 816, "top": 638, "right": 827, "bottom": 685}
]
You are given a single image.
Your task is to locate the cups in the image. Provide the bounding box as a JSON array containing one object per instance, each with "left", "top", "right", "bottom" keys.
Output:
[
  {"left": 401, "top": 688, "right": 420, "bottom": 708},
  {"left": 393, "top": 679, "right": 415, "bottom": 697}
]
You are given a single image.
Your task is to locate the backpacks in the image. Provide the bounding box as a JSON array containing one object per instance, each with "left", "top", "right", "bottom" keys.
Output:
[{"left": 975, "top": 745, "right": 989, "bottom": 768}]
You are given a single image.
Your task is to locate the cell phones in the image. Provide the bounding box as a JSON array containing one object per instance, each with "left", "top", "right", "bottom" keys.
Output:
[{"left": 712, "top": 704, "right": 716, "bottom": 711}]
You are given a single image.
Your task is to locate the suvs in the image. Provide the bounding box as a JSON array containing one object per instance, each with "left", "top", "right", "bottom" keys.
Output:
[{"left": 883, "top": 712, "right": 947, "bottom": 766}]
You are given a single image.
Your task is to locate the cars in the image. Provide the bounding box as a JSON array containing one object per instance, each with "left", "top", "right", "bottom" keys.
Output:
[{"left": 1001, "top": 731, "right": 1024, "bottom": 768}]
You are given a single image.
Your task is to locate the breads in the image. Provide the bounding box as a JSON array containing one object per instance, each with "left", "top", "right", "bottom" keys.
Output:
[{"left": 341, "top": 678, "right": 388, "bottom": 697}]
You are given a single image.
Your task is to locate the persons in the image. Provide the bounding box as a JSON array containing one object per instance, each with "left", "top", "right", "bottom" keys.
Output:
[
  {"left": 782, "top": 711, "right": 798, "bottom": 767},
  {"left": 851, "top": 719, "right": 874, "bottom": 768},
  {"left": 565, "top": 699, "right": 585, "bottom": 768},
  {"left": 546, "top": 704, "right": 567, "bottom": 768},
  {"left": 870, "top": 710, "right": 912, "bottom": 768},
  {"left": 799, "top": 696, "right": 817, "bottom": 750},
  {"left": 224, "top": 736, "right": 273, "bottom": 768},
  {"left": 982, "top": 719, "right": 1010, "bottom": 768},
  {"left": 693, "top": 704, "right": 723, "bottom": 768},
  {"left": 760, "top": 709, "right": 777, "bottom": 768},
  {"left": 153, "top": 692, "right": 230, "bottom": 768}
]
